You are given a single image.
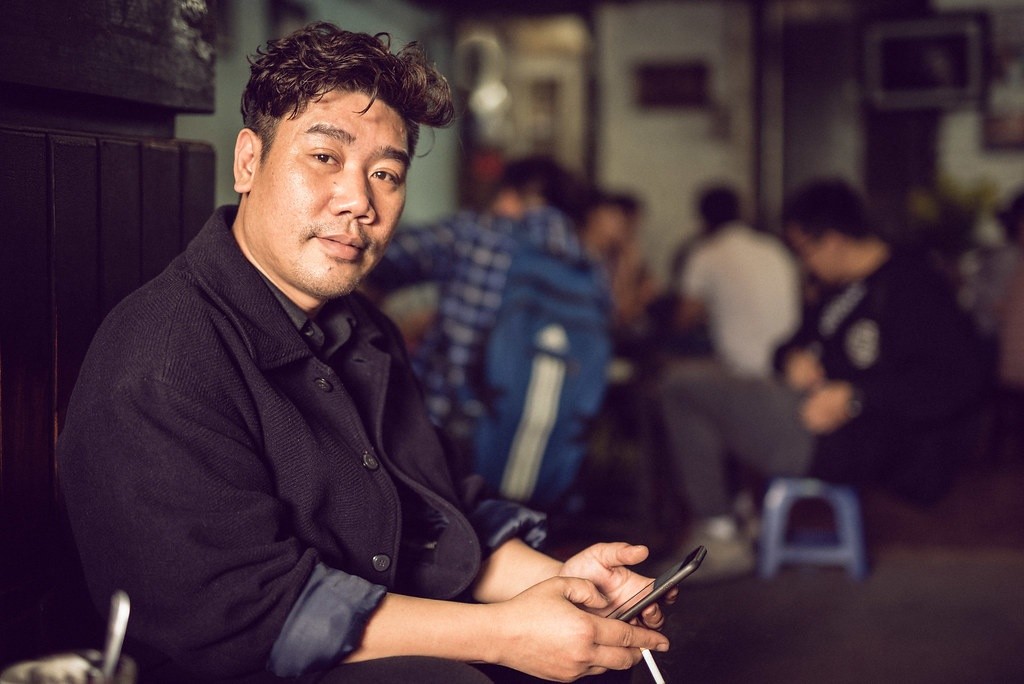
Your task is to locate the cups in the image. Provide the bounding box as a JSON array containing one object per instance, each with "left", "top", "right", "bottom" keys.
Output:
[{"left": 0, "top": 650, "right": 139, "bottom": 684}]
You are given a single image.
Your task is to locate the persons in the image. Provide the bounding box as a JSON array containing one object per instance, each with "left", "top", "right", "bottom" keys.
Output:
[
  {"left": 369, "top": 146, "right": 1024, "bottom": 587},
  {"left": 57, "top": 19, "right": 672, "bottom": 684}
]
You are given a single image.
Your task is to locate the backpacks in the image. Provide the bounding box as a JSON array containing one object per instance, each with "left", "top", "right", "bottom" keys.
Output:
[{"left": 472, "top": 249, "right": 608, "bottom": 503}]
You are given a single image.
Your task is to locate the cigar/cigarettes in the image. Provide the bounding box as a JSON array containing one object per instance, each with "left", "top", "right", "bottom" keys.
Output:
[{"left": 638, "top": 644, "right": 664, "bottom": 684}]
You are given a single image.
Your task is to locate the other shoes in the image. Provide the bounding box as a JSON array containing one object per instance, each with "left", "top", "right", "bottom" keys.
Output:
[{"left": 654, "top": 525, "right": 757, "bottom": 586}]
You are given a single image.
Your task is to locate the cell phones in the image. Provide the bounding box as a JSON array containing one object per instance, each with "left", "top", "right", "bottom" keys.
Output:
[{"left": 604, "top": 545, "right": 707, "bottom": 622}]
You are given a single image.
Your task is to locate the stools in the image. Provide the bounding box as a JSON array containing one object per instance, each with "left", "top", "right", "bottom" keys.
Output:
[{"left": 753, "top": 474, "right": 864, "bottom": 580}]
